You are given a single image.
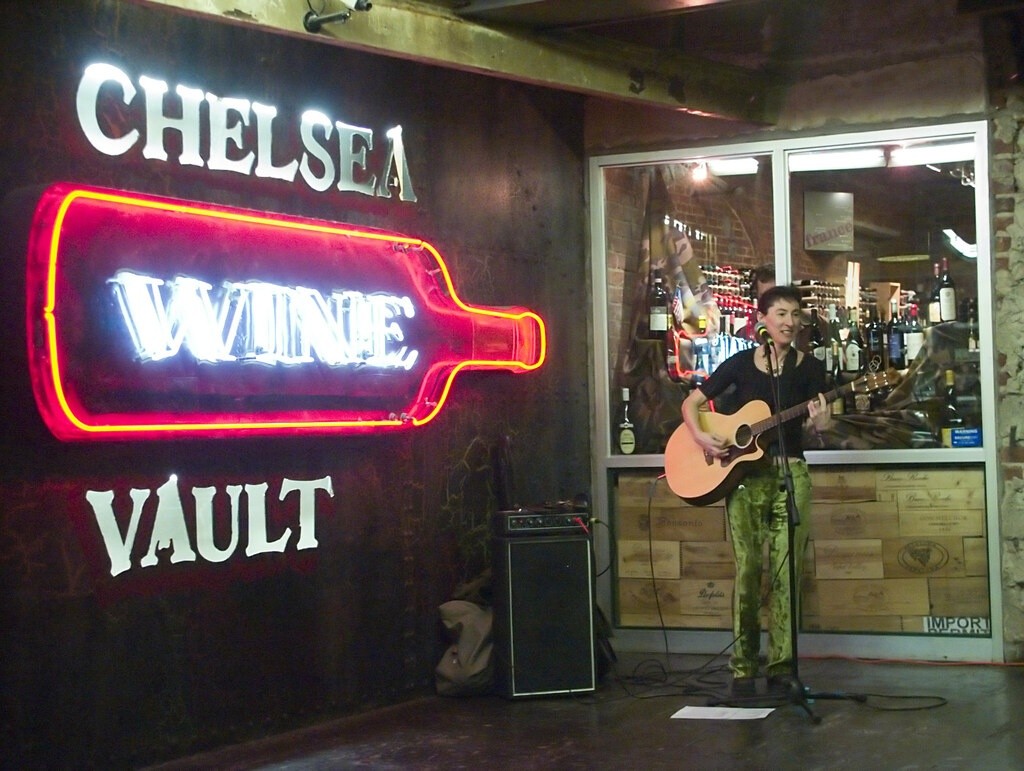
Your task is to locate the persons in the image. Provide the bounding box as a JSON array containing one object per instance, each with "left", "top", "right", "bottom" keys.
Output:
[{"left": 680, "top": 285, "right": 847, "bottom": 706}]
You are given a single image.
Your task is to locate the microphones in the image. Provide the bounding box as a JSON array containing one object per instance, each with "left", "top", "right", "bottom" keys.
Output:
[{"left": 755, "top": 329, "right": 775, "bottom": 346}]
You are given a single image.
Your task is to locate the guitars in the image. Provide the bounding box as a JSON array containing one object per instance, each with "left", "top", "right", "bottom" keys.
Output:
[{"left": 663, "top": 364, "right": 901, "bottom": 506}]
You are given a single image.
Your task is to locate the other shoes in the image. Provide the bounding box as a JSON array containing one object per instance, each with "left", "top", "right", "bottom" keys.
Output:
[
  {"left": 731, "top": 674, "right": 757, "bottom": 698},
  {"left": 766, "top": 673, "right": 798, "bottom": 694}
]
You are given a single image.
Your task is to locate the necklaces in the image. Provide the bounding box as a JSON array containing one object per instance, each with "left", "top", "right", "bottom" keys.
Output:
[{"left": 762, "top": 349, "right": 784, "bottom": 374}]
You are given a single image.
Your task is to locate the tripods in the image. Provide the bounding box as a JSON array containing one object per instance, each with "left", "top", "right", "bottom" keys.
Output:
[{"left": 704, "top": 341, "right": 867, "bottom": 726}]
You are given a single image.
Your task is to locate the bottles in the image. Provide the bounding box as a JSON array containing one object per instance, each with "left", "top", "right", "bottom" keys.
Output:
[
  {"left": 648, "top": 269, "right": 668, "bottom": 340},
  {"left": 615, "top": 388, "right": 637, "bottom": 455},
  {"left": 664, "top": 314, "right": 680, "bottom": 383},
  {"left": 694, "top": 317, "right": 711, "bottom": 385},
  {"left": 938, "top": 370, "right": 965, "bottom": 448},
  {"left": 966, "top": 317, "right": 977, "bottom": 350},
  {"left": 928, "top": 256, "right": 957, "bottom": 325},
  {"left": 809, "top": 299, "right": 924, "bottom": 414}
]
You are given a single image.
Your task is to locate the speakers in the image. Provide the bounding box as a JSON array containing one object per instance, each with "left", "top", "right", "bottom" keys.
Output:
[{"left": 491, "top": 532, "right": 597, "bottom": 699}]
